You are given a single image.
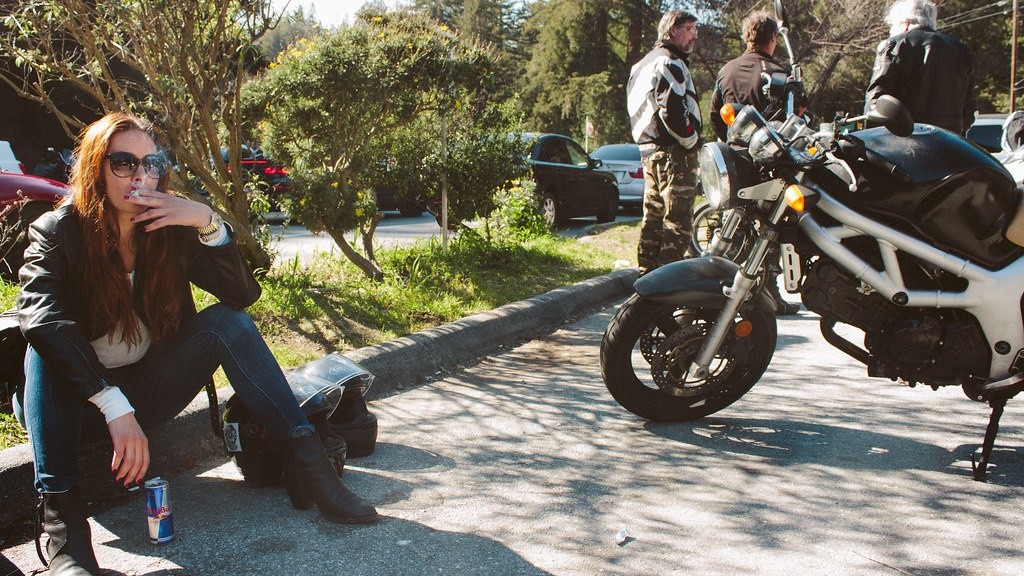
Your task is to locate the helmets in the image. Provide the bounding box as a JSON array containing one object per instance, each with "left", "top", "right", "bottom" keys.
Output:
[
  {"left": 220, "top": 371, "right": 350, "bottom": 490},
  {"left": 294, "top": 354, "right": 377, "bottom": 459}
]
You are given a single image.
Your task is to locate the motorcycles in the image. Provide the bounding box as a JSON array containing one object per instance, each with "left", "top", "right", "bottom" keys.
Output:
[{"left": 597, "top": 0, "right": 1024, "bottom": 488}]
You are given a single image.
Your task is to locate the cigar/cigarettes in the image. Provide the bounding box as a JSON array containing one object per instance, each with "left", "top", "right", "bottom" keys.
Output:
[{"left": 130, "top": 188, "right": 140, "bottom": 196}]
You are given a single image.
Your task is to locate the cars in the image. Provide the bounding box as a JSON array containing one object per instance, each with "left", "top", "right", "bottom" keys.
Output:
[
  {"left": 577, "top": 143, "right": 646, "bottom": 214},
  {"left": 0, "top": 141, "right": 68, "bottom": 285},
  {"left": 165, "top": 143, "right": 299, "bottom": 214},
  {"left": 422, "top": 130, "right": 620, "bottom": 233},
  {"left": 350, "top": 149, "right": 426, "bottom": 219},
  {"left": 964, "top": 118, "right": 1005, "bottom": 158}
]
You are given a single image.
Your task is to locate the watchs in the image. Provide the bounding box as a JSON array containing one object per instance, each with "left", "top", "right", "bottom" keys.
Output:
[{"left": 196, "top": 212, "right": 223, "bottom": 242}]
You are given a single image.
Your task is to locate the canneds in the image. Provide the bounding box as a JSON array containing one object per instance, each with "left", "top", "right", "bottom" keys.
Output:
[{"left": 144, "top": 479, "right": 175, "bottom": 545}]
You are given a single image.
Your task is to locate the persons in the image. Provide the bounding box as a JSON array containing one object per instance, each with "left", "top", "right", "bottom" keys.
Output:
[
  {"left": 864, "top": 1, "right": 979, "bottom": 136},
  {"left": 17, "top": 111, "right": 381, "bottom": 576},
  {"left": 627, "top": 8, "right": 704, "bottom": 280},
  {"left": 710, "top": 12, "right": 800, "bottom": 316}
]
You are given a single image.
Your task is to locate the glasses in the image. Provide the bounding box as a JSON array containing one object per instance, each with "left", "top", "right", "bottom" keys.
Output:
[
  {"left": 677, "top": 25, "right": 698, "bottom": 35},
  {"left": 103, "top": 152, "right": 168, "bottom": 179}
]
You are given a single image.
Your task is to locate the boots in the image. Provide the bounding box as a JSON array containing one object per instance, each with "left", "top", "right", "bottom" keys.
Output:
[
  {"left": 272, "top": 432, "right": 381, "bottom": 524},
  {"left": 34, "top": 484, "right": 101, "bottom": 576},
  {"left": 761, "top": 270, "right": 800, "bottom": 315}
]
